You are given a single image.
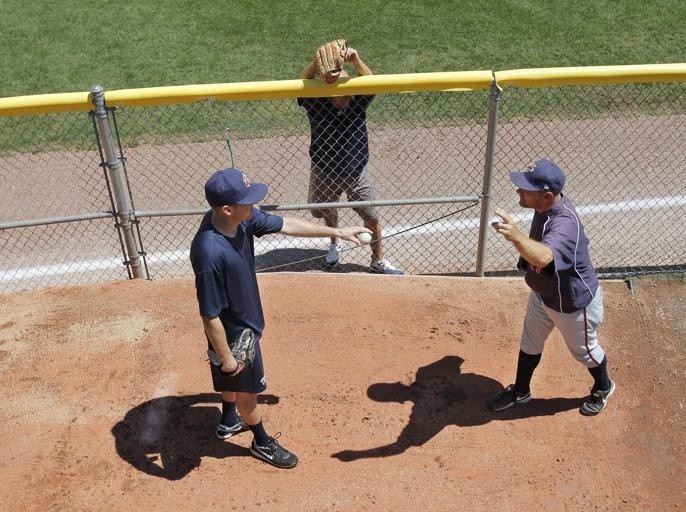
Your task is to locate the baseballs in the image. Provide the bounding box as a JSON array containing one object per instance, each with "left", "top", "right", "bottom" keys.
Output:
[{"left": 359, "top": 232, "right": 371, "bottom": 245}]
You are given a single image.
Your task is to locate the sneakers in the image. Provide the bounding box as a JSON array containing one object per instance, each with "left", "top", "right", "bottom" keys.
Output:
[
  {"left": 250, "top": 432, "right": 298, "bottom": 468},
  {"left": 488, "top": 384, "right": 531, "bottom": 411},
  {"left": 370, "top": 254, "right": 405, "bottom": 274},
  {"left": 216, "top": 416, "right": 250, "bottom": 439},
  {"left": 581, "top": 377, "right": 615, "bottom": 416},
  {"left": 324, "top": 241, "right": 342, "bottom": 268}
]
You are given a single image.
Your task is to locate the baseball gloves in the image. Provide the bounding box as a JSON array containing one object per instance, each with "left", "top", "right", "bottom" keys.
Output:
[
  {"left": 207, "top": 328, "right": 256, "bottom": 377},
  {"left": 315, "top": 39, "right": 348, "bottom": 83}
]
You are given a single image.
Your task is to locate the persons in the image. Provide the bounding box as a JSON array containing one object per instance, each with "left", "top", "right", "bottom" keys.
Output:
[
  {"left": 295, "top": 37, "right": 402, "bottom": 274},
  {"left": 490, "top": 160, "right": 616, "bottom": 415},
  {"left": 190, "top": 171, "right": 373, "bottom": 468}
]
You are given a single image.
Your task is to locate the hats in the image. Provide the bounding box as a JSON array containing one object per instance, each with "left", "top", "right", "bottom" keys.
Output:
[
  {"left": 205, "top": 169, "right": 267, "bottom": 206},
  {"left": 510, "top": 160, "right": 565, "bottom": 194}
]
